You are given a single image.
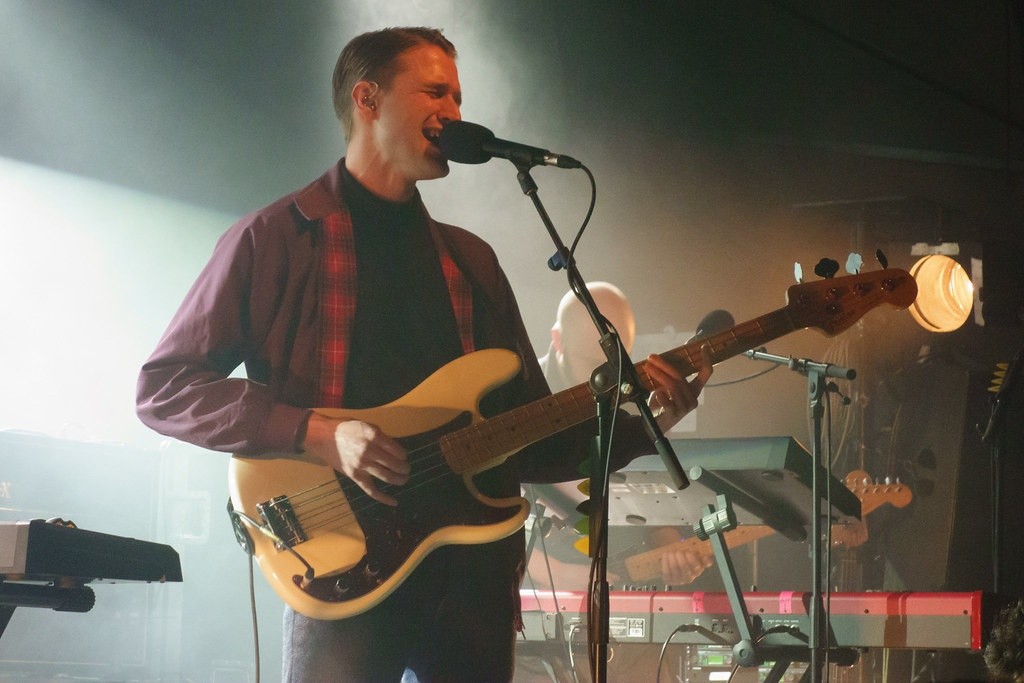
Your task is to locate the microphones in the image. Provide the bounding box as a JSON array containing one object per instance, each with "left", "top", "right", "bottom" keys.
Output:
[
  {"left": 438, "top": 119, "right": 582, "bottom": 170},
  {"left": 682, "top": 310, "right": 735, "bottom": 348}
]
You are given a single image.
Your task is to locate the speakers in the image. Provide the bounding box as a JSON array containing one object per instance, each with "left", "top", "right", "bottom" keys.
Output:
[{"left": 882, "top": 348, "right": 1024, "bottom": 601}]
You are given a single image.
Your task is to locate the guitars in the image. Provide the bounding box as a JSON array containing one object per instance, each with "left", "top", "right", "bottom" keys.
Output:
[
  {"left": 826, "top": 471, "right": 874, "bottom": 683},
  {"left": 230, "top": 247, "right": 919, "bottom": 623},
  {"left": 541, "top": 475, "right": 914, "bottom": 591}
]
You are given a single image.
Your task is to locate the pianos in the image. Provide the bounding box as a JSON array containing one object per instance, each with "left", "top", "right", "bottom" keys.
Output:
[{"left": 1, "top": 516, "right": 185, "bottom": 639}]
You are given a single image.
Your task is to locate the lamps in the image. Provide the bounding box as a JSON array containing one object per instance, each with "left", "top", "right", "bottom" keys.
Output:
[{"left": 906, "top": 254, "right": 974, "bottom": 334}]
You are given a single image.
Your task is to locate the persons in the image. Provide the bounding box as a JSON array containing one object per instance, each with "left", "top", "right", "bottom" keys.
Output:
[
  {"left": 511, "top": 280, "right": 713, "bottom": 683},
  {"left": 134, "top": 25, "right": 714, "bottom": 683}
]
